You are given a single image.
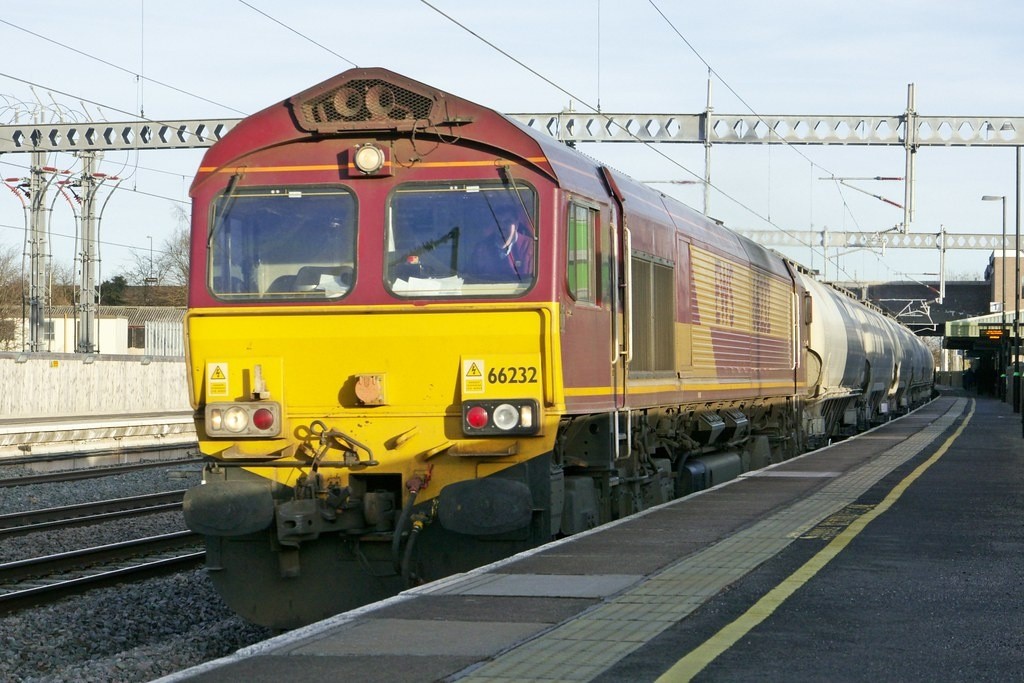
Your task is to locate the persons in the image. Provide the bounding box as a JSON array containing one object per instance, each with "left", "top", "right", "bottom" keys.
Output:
[
  {"left": 987, "top": 365, "right": 997, "bottom": 395},
  {"left": 464, "top": 205, "right": 533, "bottom": 275},
  {"left": 965, "top": 368, "right": 974, "bottom": 391}
]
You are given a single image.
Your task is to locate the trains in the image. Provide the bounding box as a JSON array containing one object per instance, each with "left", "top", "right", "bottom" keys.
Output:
[{"left": 181, "top": 67, "right": 934, "bottom": 632}]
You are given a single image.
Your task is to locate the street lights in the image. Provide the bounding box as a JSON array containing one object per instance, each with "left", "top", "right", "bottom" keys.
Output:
[
  {"left": 147, "top": 235, "right": 152, "bottom": 287},
  {"left": 980, "top": 195, "right": 1006, "bottom": 328}
]
